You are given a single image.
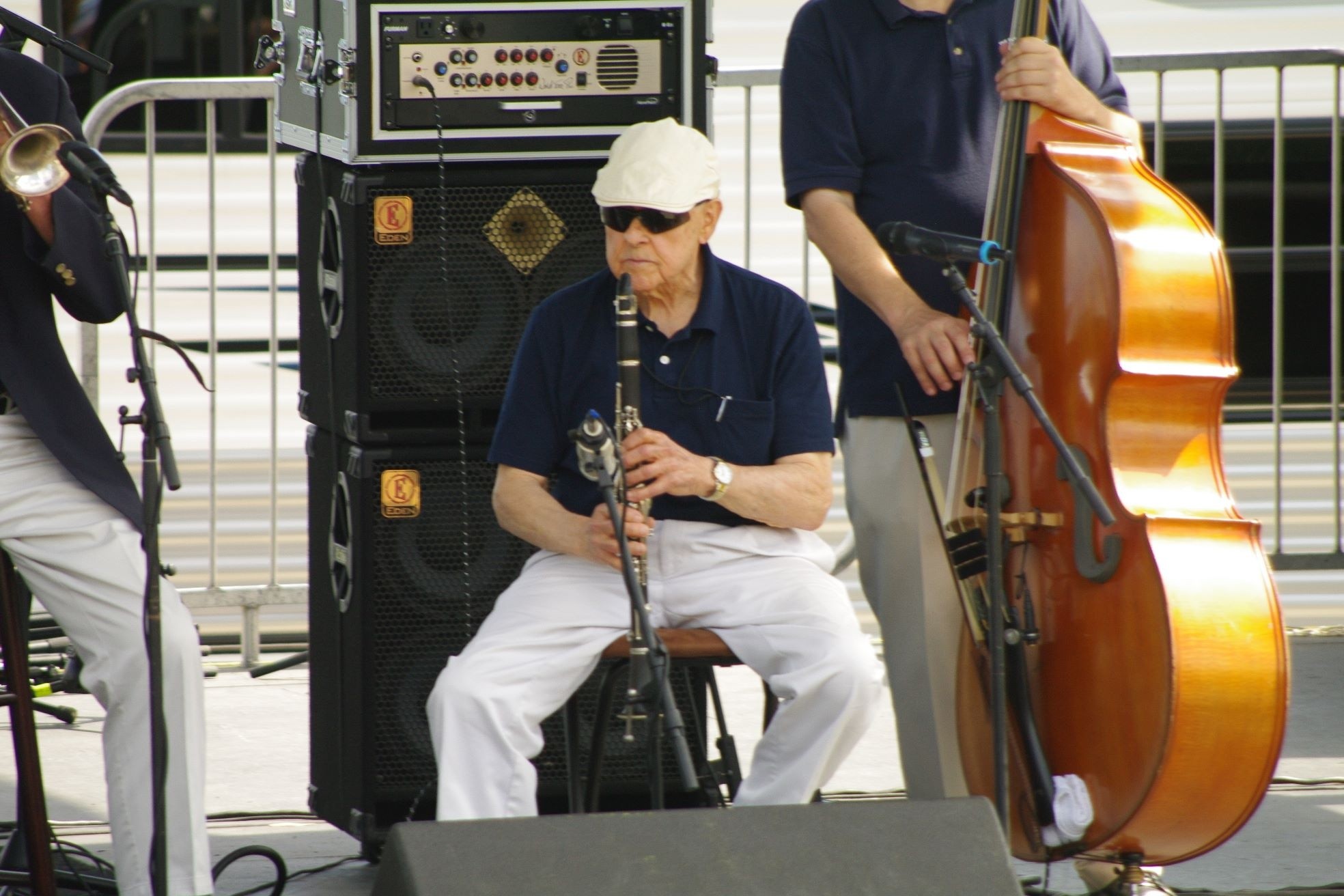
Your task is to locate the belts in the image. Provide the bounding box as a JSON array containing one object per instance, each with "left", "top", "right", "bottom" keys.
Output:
[{"left": 0, "top": 397, "right": 16, "bottom": 415}]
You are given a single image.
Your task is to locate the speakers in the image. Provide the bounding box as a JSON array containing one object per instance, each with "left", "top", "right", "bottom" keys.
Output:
[
  {"left": 292, "top": 149, "right": 706, "bottom": 838},
  {"left": 374, "top": 796, "right": 1024, "bottom": 896}
]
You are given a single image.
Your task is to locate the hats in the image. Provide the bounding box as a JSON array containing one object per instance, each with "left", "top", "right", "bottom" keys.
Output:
[{"left": 591, "top": 116, "right": 720, "bottom": 213}]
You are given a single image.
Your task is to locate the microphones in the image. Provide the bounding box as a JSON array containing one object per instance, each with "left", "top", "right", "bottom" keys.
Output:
[
  {"left": 57, "top": 141, "right": 134, "bottom": 206},
  {"left": 874, "top": 220, "right": 1010, "bottom": 266},
  {"left": 577, "top": 410, "right": 617, "bottom": 482}
]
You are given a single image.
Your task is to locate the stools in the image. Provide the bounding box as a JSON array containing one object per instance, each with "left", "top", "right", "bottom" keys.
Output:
[{"left": 580, "top": 629, "right": 822, "bottom": 813}]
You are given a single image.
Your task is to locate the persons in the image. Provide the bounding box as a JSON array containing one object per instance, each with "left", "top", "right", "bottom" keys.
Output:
[
  {"left": 781, "top": 0, "right": 1175, "bottom": 896},
  {"left": 0, "top": 50, "right": 215, "bottom": 896},
  {"left": 426, "top": 119, "right": 888, "bottom": 822}
]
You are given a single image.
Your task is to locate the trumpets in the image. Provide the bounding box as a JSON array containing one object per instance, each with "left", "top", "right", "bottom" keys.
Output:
[{"left": 0, "top": 90, "right": 78, "bottom": 198}]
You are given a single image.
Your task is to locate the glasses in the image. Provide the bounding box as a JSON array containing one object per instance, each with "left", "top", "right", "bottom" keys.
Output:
[{"left": 599, "top": 199, "right": 711, "bottom": 235}]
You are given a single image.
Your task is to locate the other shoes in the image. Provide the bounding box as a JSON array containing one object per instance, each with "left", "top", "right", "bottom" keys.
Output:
[{"left": 1077, "top": 863, "right": 1174, "bottom": 896}]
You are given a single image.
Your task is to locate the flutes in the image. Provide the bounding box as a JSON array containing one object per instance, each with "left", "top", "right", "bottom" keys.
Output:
[{"left": 609, "top": 265, "right": 664, "bottom": 720}]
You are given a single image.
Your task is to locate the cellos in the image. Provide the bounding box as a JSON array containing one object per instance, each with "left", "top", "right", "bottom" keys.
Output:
[{"left": 944, "top": 0, "right": 1299, "bottom": 896}]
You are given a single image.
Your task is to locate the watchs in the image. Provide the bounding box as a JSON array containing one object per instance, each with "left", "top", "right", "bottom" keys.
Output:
[{"left": 697, "top": 456, "right": 732, "bottom": 501}]
